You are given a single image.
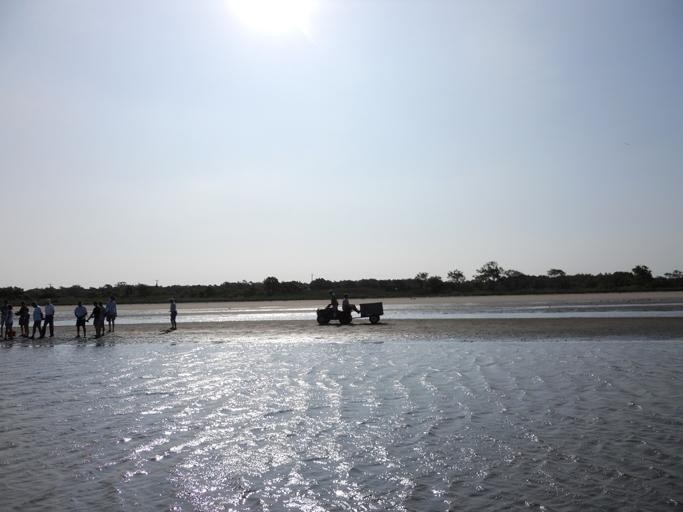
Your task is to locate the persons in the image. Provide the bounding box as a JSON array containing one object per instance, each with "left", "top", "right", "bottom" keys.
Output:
[
  {"left": 27, "top": 302, "right": 43, "bottom": 339},
  {"left": 73, "top": 301, "right": 87, "bottom": 338},
  {"left": 85, "top": 301, "right": 100, "bottom": 338},
  {"left": 328, "top": 289, "right": 338, "bottom": 309},
  {"left": 341, "top": 293, "right": 360, "bottom": 314},
  {"left": 105, "top": 294, "right": 116, "bottom": 333},
  {"left": 0, "top": 299, "right": 30, "bottom": 341},
  {"left": 98, "top": 302, "right": 106, "bottom": 336},
  {"left": 39, "top": 298, "right": 54, "bottom": 337},
  {"left": 167, "top": 296, "right": 176, "bottom": 330}
]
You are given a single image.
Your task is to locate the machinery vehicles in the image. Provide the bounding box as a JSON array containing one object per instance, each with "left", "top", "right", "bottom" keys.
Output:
[{"left": 316, "top": 302, "right": 353, "bottom": 326}]
